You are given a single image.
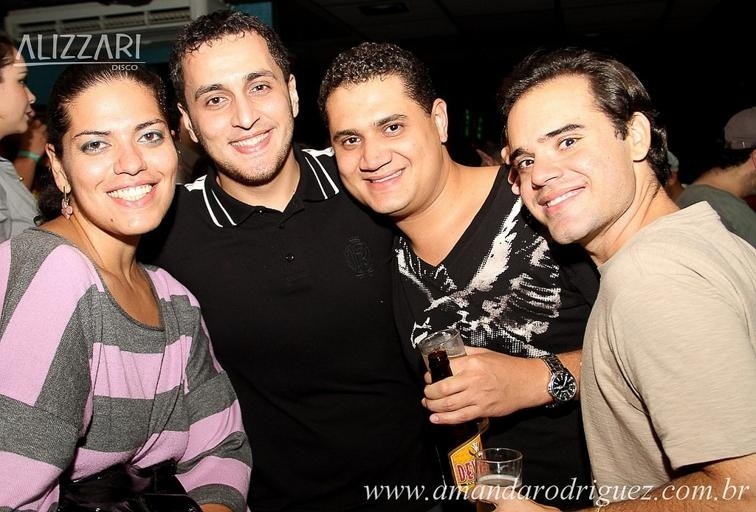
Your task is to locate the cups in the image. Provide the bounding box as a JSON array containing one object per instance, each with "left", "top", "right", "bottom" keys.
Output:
[
  {"left": 418, "top": 328, "right": 467, "bottom": 372},
  {"left": 474, "top": 447, "right": 524, "bottom": 512}
]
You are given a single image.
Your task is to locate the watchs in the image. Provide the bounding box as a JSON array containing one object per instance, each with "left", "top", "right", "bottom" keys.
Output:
[{"left": 540, "top": 351, "right": 579, "bottom": 415}]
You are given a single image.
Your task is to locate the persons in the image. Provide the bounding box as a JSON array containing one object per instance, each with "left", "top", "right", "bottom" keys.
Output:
[
  {"left": 133, "top": 8, "right": 454, "bottom": 508},
  {"left": 450, "top": 52, "right": 756, "bottom": 510},
  {"left": 0, "top": 30, "right": 756, "bottom": 251},
  {"left": 312, "top": 38, "right": 606, "bottom": 510},
  {"left": 0, "top": 57, "right": 259, "bottom": 512}
]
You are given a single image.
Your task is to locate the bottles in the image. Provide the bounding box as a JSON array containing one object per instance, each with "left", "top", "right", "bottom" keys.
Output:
[{"left": 423, "top": 350, "right": 490, "bottom": 498}]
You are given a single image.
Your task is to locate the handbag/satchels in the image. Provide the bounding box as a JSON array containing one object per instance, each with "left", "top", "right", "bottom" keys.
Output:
[{"left": 57, "top": 459, "right": 201, "bottom": 512}]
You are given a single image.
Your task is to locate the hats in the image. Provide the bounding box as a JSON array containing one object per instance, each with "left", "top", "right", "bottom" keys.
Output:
[{"left": 724, "top": 106, "right": 756, "bottom": 150}]
